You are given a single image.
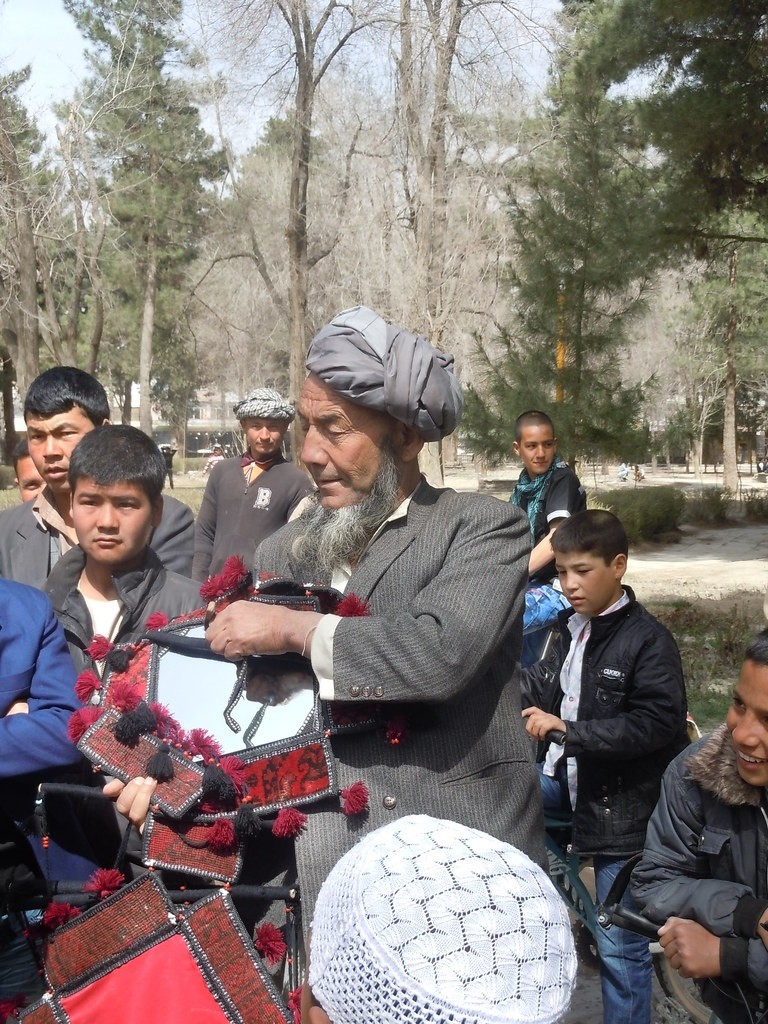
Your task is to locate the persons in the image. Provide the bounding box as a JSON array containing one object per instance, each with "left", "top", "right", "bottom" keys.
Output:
[
  {"left": 102, "top": 306, "right": 550, "bottom": 968},
  {"left": 12, "top": 439, "right": 46, "bottom": 503},
  {"left": 0, "top": 576, "right": 134, "bottom": 1004},
  {"left": 303, "top": 815, "right": 580, "bottom": 1024},
  {"left": 508, "top": 410, "right": 589, "bottom": 676},
  {"left": 627, "top": 624, "right": 768, "bottom": 1024},
  {"left": 517, "top": 509, "right": 693, "bottom": 1023},
  {"left": 190, "top": 387, "right": 314, "bottom": 584},
  {"left": 0, "top": 366, "right": 197, "bottom": 589},
  {"left": 41, "top": 424, "right": 270, "bottom": 931},
  {"left": 161, "top": 446, "right": 177, "bottom": 489},
  {"left": 202, "top": 448, "right": 225, "bottom": 479}
]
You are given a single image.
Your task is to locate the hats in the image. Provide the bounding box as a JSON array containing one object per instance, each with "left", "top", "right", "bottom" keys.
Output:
[
  {"left": 304, "top": 305, "right": 462, "bottom": 442},
  {"left": 234, "top": 387, "right": 298, "bottom": 425},
  {"left": 308, "top": 814, "right": 576, "bottom": 1024}
]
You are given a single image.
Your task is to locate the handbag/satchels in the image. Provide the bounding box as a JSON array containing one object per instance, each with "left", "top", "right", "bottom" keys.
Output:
[{"left": 523, "top": 583, "right": 572, "bottom": 635}]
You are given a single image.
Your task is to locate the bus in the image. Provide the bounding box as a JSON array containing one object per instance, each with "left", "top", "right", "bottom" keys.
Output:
[{"left": 153, "top": 426, "right": 291, "bottom": 473}]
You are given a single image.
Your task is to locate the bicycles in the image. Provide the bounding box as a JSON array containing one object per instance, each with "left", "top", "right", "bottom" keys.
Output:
[{"left": 521, "top": 712, "right": 715, "bottom": 1023}]
